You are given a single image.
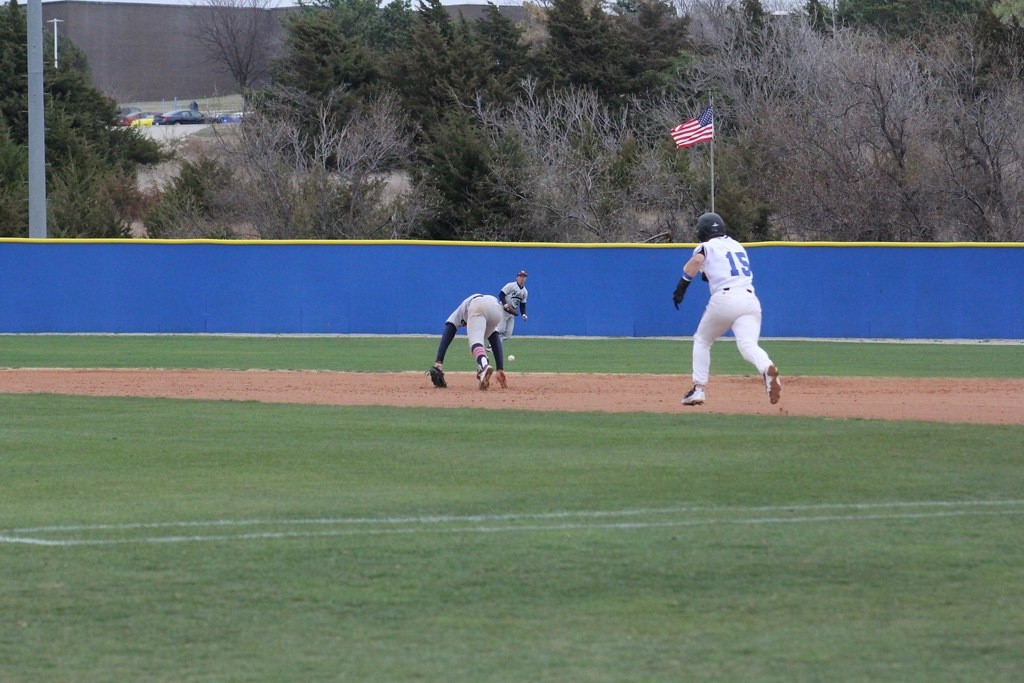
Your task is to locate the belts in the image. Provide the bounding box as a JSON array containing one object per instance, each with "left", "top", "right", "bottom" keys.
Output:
[{"left": 724, "top": 288, "right": 752, "bottom": 293}]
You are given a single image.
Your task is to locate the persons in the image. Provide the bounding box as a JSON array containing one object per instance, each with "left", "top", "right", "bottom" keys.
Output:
[
  {"left": 673, "top": 213, "right": 782, "bottom": 404},
  {"left": 433, "top": 293, "right": 507, "bottom": 390},
  {"left": 485, "top": 270, "right": 528, "bottom": 352}
]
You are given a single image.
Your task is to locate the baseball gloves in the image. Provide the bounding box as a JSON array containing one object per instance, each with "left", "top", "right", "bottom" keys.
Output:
[
  {"left": 429, "top": 366, "right": 446, "bottom": 388},
  {"left": 505, "top": 305, "right": 518, "bottom": 316}
]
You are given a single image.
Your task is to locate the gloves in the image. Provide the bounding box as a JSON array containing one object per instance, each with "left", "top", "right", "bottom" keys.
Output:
[{"left": 673, "top": 279, "right": 691, "bottom": 304}]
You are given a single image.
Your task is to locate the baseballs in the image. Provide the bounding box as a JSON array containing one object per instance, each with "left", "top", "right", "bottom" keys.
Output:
[{"left": 507, "top": 354, "right": 513, "bottom": 361}]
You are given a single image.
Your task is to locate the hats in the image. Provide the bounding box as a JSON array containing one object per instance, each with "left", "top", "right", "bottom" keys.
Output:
[{"left": 517, "top": 270, "right": 528, "bottom": 277}]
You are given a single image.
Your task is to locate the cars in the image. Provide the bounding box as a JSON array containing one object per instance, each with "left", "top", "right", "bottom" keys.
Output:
[{"left": 110, "top": 105, "right": 255, "bottom": 127}]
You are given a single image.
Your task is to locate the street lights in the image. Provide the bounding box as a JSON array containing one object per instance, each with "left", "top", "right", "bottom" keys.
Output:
[{"left": 45, "top": 18, "right": 65, "bottom": 69}]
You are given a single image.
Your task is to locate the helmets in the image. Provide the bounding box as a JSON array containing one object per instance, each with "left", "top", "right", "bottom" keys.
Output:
[{"left": 696, "top": 213, "right": 725, "bottom": 240}]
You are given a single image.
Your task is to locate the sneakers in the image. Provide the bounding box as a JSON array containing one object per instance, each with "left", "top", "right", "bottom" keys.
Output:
[
  {"left": 681, "top": 385, "right": 705, "bottom": 406},
  {"left": 486, "top": 344, "right": 492, "bottom": 352},
  {"left": 477, "top": 365, "right": 493, "bottom": 390},
  {"left": 764, "top": 365, "right": 782, "bottom": 404}
]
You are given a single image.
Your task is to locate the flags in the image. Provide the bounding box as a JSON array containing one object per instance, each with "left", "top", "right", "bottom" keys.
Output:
[{"left": 670, "top": 105, "right": 713, "bottom": 149}]
